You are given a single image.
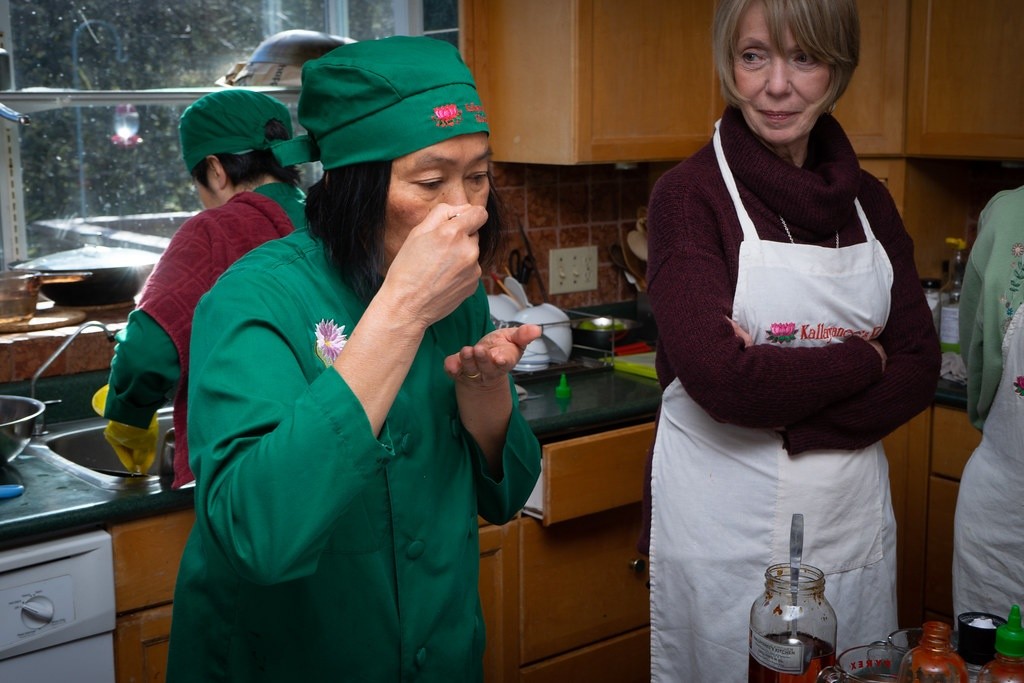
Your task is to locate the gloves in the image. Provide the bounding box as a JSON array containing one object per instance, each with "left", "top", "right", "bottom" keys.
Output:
[{"left": 91, "top": 382, "right": 161, "bottom": 484}]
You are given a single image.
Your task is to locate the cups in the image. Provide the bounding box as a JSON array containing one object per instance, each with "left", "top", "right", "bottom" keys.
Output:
[
  {"left": 816, "top": 645, "right": 908, "bottom": 683},
  {"left": 957, "top": 612, "right": 1007, "bottom": 672},
  {"left": 868, "top": 628, "right": 957, "bottom": 656}
]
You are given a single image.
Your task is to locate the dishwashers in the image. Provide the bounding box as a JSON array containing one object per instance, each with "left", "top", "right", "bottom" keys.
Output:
[{"left": 0, "top": 530, "right": 119, "bottom": 683}]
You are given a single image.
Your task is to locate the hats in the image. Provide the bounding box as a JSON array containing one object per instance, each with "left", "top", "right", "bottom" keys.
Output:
[
  {"left": 178, "top": 90, "right": 292, "bottom": 174},
  {"left": 301, "top": 35, "right": 490, "bottom": 171}
]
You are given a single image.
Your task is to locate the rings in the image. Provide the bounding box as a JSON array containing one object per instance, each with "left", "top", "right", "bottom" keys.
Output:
[{"left": 465, "top": 370, "right": 481, "bottom": 377}]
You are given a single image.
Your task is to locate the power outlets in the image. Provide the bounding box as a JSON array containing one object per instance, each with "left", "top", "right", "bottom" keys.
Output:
[{"left": 550, "top": 245, "right": 598, "bottom": 294}]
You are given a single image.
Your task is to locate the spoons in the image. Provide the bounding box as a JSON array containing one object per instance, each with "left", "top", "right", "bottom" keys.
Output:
[
  {"left": 607, "top": 242, "right": 630, "bottom": 272},
  {"left": 781, "top": 512, "right": 811, "bottom": 674}
]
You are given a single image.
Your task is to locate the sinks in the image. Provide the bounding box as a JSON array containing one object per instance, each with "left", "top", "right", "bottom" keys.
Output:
[{"left": 45, "top": 411, "right": 175, "bottom": 479}]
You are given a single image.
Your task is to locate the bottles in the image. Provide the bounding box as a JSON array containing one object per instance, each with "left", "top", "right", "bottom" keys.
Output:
[
  {"left": 895, "top": 621, "right": 970, "bottom": 683},
  {"left": 940, "top": 239, "right": 972, "bottom": 355},
  {"left": 976, "top": 605, "right": 1024, "bottom": 683},
  {"left": 747, "top": 564, "right": 836, "bottom": 683},
  {"left": 919, "top": 277, "right": 941, "bottom": 337}
]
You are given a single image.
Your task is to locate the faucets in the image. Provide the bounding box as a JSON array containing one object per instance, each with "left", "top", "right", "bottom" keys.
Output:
[{"left": 29, "top": 320, "right": 115, "bottom": 436}]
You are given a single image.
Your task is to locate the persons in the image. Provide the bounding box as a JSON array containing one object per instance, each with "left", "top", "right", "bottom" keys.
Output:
[
  {"left": 953, "top": 183, "right": 1024, "bottom": 629},
  {"left": 102, "top": 87, "right": 307, "bottom": 488},
  {"left": 635, "top": 0, "right": 942, "bottom": 683},
  {"left": 166, "top": 33, "right": 543, "bottom": 683}
]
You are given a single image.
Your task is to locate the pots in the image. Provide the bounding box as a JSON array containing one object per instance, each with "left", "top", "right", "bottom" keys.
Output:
[
  {"left": 8, "top": 247, "right": 162, "bottom": 307},
  {"left": 0, "top": 270, "right": 94, "bottom": 323},
  {"left": 249, "top": 28, "right": 361, "bottom": 65}
]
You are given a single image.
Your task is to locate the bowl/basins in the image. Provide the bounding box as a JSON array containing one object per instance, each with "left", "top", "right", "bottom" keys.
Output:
[
  {"left": 571, "top": 318, "right": 639, "bottom": 349},
  {"left": 0, "top": 395, "right": 47, "bottom": 465},
  {"left": 484, "top": 293, "right": 573, "bottom": 373}
]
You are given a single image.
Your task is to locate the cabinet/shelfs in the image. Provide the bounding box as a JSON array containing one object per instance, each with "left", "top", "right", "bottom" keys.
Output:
[
  {"left": 459, "top": 0, "right": 1024, "bottom": 164},
  {"left": 108, "top": 399, "right": 983, "bottom": 683}
]
